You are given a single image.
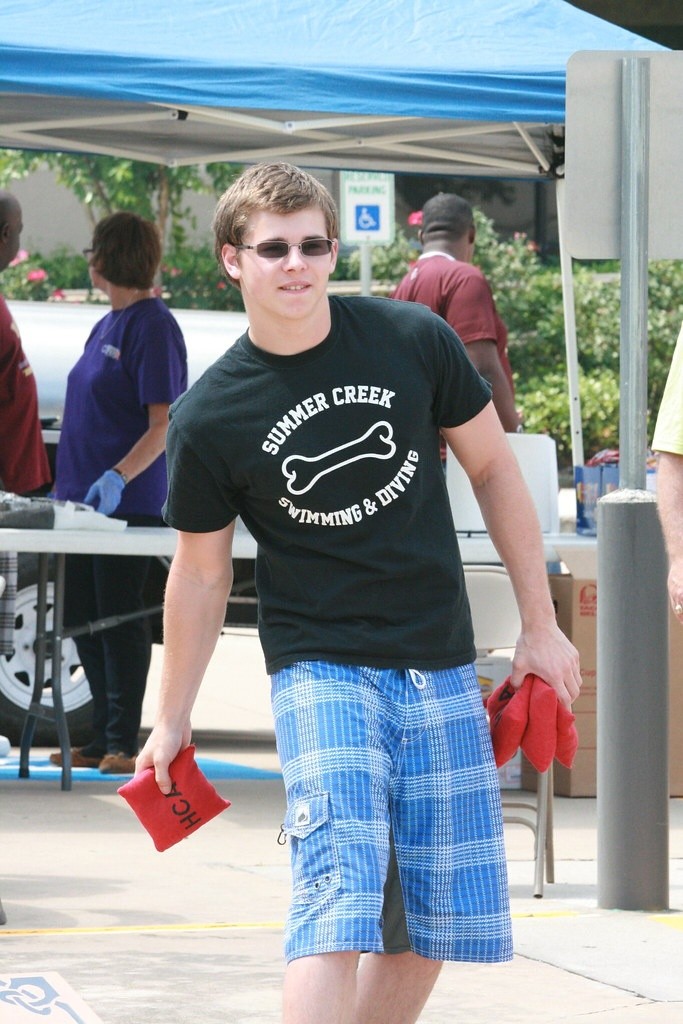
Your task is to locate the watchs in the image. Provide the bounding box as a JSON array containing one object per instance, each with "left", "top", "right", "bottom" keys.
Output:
[{"left": 113, "top": 468, "right": 128, "bottom": 483}]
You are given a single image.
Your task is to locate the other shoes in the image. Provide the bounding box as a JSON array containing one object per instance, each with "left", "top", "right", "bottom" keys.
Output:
[
  {"left": 49, "top": 749, "right": 104, "bottom": 767},
  {"left": 99, "top": 753, "right": 139, "bottom": 773}
]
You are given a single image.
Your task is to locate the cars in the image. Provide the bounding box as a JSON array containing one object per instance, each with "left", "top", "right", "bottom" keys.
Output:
[{"left": 3, "top": 298, "right": 275, "bottom": 756}]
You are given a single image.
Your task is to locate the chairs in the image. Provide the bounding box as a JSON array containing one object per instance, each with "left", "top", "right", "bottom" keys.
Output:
[{"left": 458, "top": 565, "right": 558, "bottom": 902}]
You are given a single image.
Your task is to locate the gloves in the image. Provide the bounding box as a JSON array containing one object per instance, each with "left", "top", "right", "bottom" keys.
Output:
[{"left": 83, "top": 469, "right": 125, "bottom": 516}]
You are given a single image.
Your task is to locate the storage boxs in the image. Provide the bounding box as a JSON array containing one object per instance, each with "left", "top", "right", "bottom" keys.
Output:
[{"left": 546, "top": 547, "right": 683, "bottom": 798}]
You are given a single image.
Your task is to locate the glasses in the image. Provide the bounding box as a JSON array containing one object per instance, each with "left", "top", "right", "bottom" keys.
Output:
[{"left": 234, "top": 239, "right": 334, "bottom": 259}]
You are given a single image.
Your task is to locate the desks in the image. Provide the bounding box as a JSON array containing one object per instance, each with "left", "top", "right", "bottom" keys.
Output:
[{"left": 0, "top": 527, "right": 596, "bottom": 791}]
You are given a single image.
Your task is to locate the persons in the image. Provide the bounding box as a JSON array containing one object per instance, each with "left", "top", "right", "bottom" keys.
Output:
[
  {"left": 135, "top": 159, "right": 583, "bottom": 1022},
  {"left": 49, "top": 212, "right": 188, "bottom": 772},
  {"left": 652, "top": 321, "right": 682, "bottom": 622},
  {"left": 0, "top": 193, "right": 53, "bottom": 697},
  {"left": 392, "top": 191, "right": 520, "bottom": 479}
]
